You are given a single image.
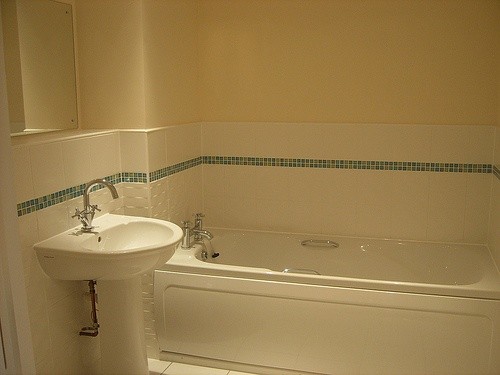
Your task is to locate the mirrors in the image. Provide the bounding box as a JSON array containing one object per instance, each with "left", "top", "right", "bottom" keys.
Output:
[{"left": 1, "top": 1, "right": 79, "bottom": 138}]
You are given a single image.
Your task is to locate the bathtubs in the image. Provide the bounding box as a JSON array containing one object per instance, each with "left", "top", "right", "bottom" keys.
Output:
[{"left": 153, "top": 227, "right": 498, "bottom": 374}]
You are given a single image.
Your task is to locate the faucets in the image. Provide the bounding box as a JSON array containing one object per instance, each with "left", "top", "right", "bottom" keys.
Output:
[
  {"left": 81, "top": 179, "right": 119, "bottom": 232},
  {"left": 193, "top": 229, "right": 219, "bottom": 259}
]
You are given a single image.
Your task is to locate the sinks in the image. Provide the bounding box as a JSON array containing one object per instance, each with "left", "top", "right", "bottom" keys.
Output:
[{"left": 33, "top": 213, "right": 183, "bottom": 281}]
